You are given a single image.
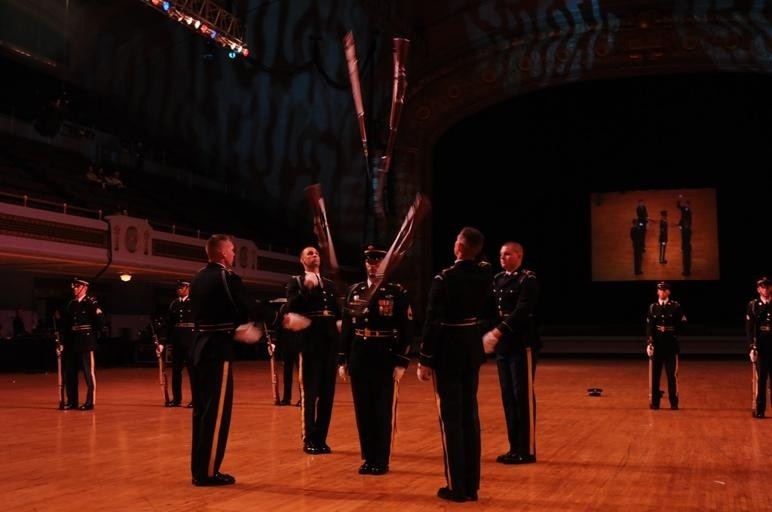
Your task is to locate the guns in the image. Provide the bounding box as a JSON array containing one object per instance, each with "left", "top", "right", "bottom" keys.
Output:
[
  {"left": 342, "top": 30, "right": 370, "bottom": 177},
  {"left": 356, "top": 191, "right": 431, "bottom": 305},
  {"left": 752, "top": 337, "right": 759, "bottom": 418},
  {"left": 383, "top": 38, "right": 409, "bottom": 173},
  {"left": 647, "top": 335, "right": 655, "bottom": 408},
  {"left": 150, "top": 322, "right": 169, "bottom": 405},
  {"left": 53, "top": 318, "right": 65, "bottom": 410},
  {"left": 306, "top": 181, "right": 343, "bottom": 313},
  {"left": 263, "top": 322, "right": 280, "bottom": 405}
]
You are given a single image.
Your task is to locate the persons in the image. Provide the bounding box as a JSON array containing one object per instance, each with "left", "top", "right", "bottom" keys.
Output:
[
  {"left": 273, "top": 281, "right": 302, "bottom": 407},
  {"left": 11, "top": 308, "right": 28, "bottom": 339},
  {"left": 745, "top": 275, "right": 772, "bottom": 419},
  {"left": 185, "top": 233, "right": 314, "bottom": 487},
  {"left": 416, "top": 226, "right": 498, "bottom": 504},
  {"left": 481, "top": 239, "right": 546, "bottom": 466},
  {"left": 286, "top": 244, "right": 346, "bottom": 455},
  {"left": 160, "top": 277, "right": 200, "bottom": 407},
  {"left": 336, "top": 240, "right": 416, "bottom": 474},
  {"left": 644, "top": 280, "right": 687, "bottom": 411},
  {"left": 628, "top": 192, "right": 695, "bottom": 276},
  {"left": 55, "top": 277, "right": 106, "bottom": 410}
]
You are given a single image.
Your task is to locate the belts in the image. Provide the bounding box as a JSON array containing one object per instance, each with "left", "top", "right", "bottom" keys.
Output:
[
  {"left": 71, "top": 324, "right": 94, "bottom": 332},
  {"left": 174, "top": 321, "right": 196, "bottom": 328},
  {"left": 440, "top": 316, "right": 479, "bottom": 328},
  {"left": 655, "top": 323, "right": 676, "bottom": 332},
  {"left": 498, "top": 310, "right": 512, "bottom": 317},
  {"left": 196, "top": 320, "right": 237, "bottom": 333},
  {"left": 756, "top": 325, "right": 771, "bottom": 332},
  {"left": 353, "top": 328, "right": 401, "bottom": 340},
  {"left": 306, "top": 309, "right": 338, "bottom": 319}
]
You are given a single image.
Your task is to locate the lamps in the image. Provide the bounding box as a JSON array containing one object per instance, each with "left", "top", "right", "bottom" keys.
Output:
[{"left": 146, "top": 0, "right": 250, "bottom": 57}]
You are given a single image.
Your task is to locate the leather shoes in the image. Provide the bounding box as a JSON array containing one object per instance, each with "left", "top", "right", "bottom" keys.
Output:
[
  {"left": 279, "top": 400, "right": 290, "bottom": 406},
  {"left": 495, "top": 449, "right": 537, "bottom": 464},
  {"left": 63, "top": 402, "right": 78, "bottom": 409},
  {"left": 166, "top": 401, "right": 182, "bottom": 407},
  {"left": 358, "top": 458, "right": 390, "bottom": 474},
  {"left": 296, "top": 400, "right": 303, "bottom": 407},
  {"left": 319, "top": 441, "right": 331, "bottom": 455},
  {"left": 188, "top": 401, "right": 194, "bottom": 407},
  {"left": 468, "top": 488, "right": 479, "bottom": 501},
  {"left": 190, "top": 471, "right": 235, "bottom": 487},
  {"left": 80, "top": 403, "right": 95, "bottom": 410},
  {"left": 303, "top": 442, "right": 319, "bottom": 455},
  {"left": 437, "top": 484, "right": 468, "bottom": 503}
]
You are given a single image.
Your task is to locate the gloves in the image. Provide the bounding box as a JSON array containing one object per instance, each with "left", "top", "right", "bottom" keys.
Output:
[
  {"left": 392, "top": 365, "right": 406, "bottom": 383},
  {"left": 304, "top": 272, "right": 320, "bottom": 286},
  {"left": 56, "top": 344, "right": 63, "bottom": 357},
  {"left": 482, "top": 331, "right": 499, "bottom": 354},
  {"left": 155, "top": 344, "right": 163, "bottom": 358},
  {"left": 284, "top": 313, "right": 312, "bottom": 332},
  {"left": 647, "top": 344, "right": 654, "bottom": 357},
  {"left": 748, "top": 349, "right": 759, "bottom": 363},
  {"left": 417, "top": 363, "right": 431, "bottom": 383},
  {"left": 337, "top": 366, "right": 347, "bottom": 382},
  {"left": 235, "top": 321, "right": 262, "bottom": 345}
]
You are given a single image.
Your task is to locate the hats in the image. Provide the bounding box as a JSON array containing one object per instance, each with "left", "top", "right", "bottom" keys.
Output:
[
  {"left": 588, "top": 388, "right": 603, "bottom": 396},
  {"left": 71, "top": 275, "right": 91, "bottom": 287},
  {"left": 175, "top": 278, "right": 193, "bottom": 288},
  {"left": 656, "top": 281, "right": 671, "bottom": 291},
  {"left": 756, "top": 277, "right": 772, "bottom": 288},
  {"left": 363, "top": 243, "right": 389, "bottom": 263}
]
